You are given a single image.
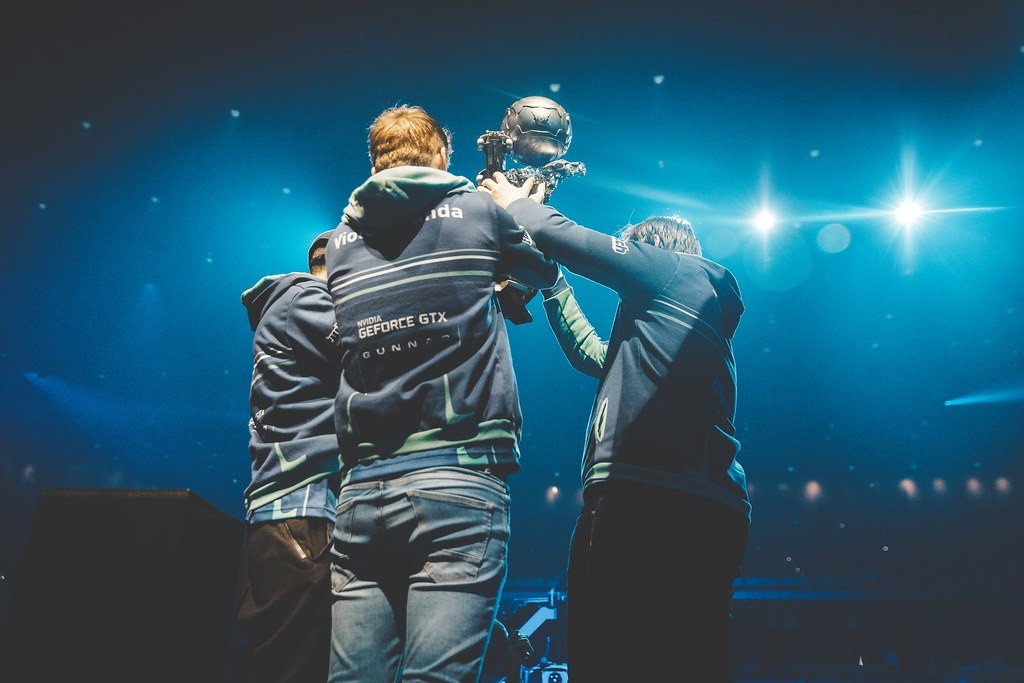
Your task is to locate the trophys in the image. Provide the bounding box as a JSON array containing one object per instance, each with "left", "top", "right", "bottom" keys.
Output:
[{"left": 476, "top": 96, "right": 585, "bottom": 204}]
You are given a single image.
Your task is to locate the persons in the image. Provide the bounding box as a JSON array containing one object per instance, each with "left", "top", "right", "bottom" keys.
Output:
[
  {"left": 477, "top": 171, "right": 751, "bottom": 683},
  {"left": 327, "top": 106, "right": 558, "bottom": 683},
  {"left": 235, "top": 229, "right": 338, "bottom": 683}
]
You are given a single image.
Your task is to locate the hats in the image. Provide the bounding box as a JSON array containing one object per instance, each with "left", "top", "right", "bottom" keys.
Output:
[{"left": 308, "top": 228, "right": 334, "bottom": 267}]
